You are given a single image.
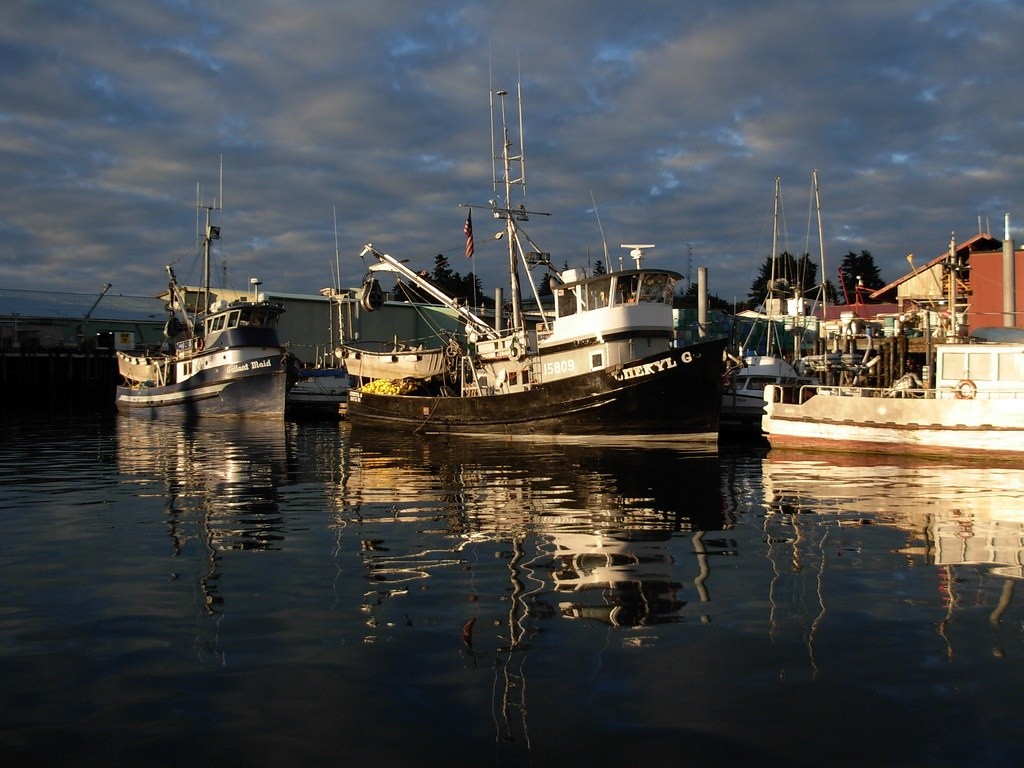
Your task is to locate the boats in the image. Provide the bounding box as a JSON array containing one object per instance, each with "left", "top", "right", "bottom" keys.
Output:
[
  {"left": 287, "top": 366, "right": 354, "bottom": 403},
  {"left": 115, "top": 153, "right": 302, "bottom": 426},
  {"left": 116, "top": 350, "right": 171, "bottom": 385},
  {"left": 335, "top": 335, "right": 450, "bottom": 382},
  {"left": 345, "top": 42, "right": 728, "bottom": 459},
  {"left": 761, "top": 326, "right": 1024, "bottom": 459}
]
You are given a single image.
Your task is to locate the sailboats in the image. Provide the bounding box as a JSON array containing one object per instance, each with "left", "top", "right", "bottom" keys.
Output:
[{"left": 720, "top": 169, "right": 894, "bottom": 417}]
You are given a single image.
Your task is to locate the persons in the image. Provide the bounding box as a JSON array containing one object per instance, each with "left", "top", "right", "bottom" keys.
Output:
[{"left": 849, "top": 369, "right": 869, "bottom": 386}]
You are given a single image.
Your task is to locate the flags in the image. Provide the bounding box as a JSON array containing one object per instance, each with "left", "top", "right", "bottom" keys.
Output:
[{"left": 464, "top": 213, "right": 474, "bottom": 257}]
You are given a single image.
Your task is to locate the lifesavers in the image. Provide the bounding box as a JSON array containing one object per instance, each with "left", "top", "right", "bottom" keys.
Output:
[
  {"left": 197, "top": 339, "right": 204, "bottom": 350},
  {"left": 954, "top": 380, "right": 976, "bottom": 399},
  {"left": 508, "top": 342, "right": 521, "bottom": 361}
]
